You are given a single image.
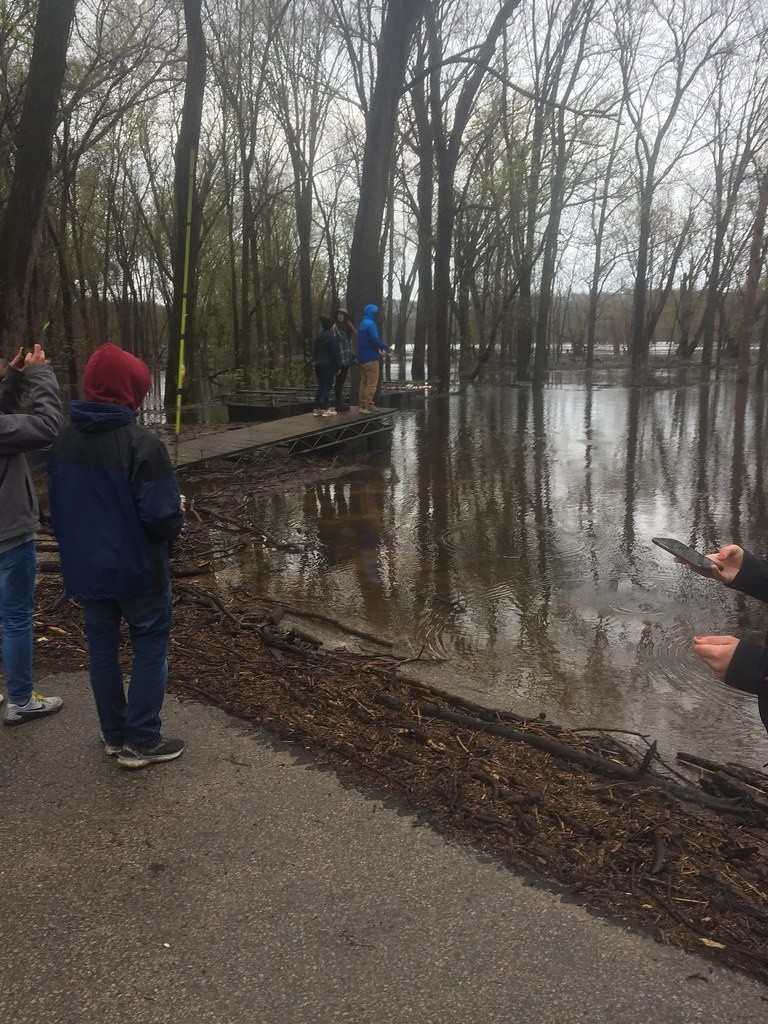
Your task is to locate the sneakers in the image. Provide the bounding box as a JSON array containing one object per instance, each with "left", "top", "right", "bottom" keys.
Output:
[
  {"left": 117, "top": 737, "right": 185, "bottom": 767},
  {"left": 313, "top": 408, "right": 322, "bottom": 416},
  {"left": 322, "top": 409, "right": 337, "bottom": 417},
  {"left": 4, "top": 691, "right": 63, "bottom": 726},
  {"left": 99, "top": 727, "right": 122, "bottom": 756}
]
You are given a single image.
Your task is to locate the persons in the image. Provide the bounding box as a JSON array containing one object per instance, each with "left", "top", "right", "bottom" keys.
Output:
[
  {"left": 46, "top": 342, "right": 187, "bottom": 769},
  {"left": 330, "top": 305, "right": 358, "bottom": 411},
  {"left": 0, "top": 342, "right": 65, "bottom": 725},
  {"left": 312, "top": 315, "right": 342, "bottom": 418},
  {"left": 673, "top": 544, "right": 768, "bottom": 734},
  {"left": 358, "top": 303, "right": 394, "bottom": 414}
]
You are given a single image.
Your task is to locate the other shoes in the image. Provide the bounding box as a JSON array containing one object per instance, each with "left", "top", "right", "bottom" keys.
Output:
[
  {"left": 335, "top": 401, "right": 351, "bottom": 406},
  {"left": 367, "top": 404, "right": 380, "bottom": 412},
  {"left": 358, "top": 407, "right": 373, "bottom": 414},
  {"left": 335, "top": 404, "right": 351, "bottom": 410}
]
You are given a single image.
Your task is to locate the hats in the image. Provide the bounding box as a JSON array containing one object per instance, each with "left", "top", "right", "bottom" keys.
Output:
[
  {"left": 337, "top": 307, "right": 348, "bottom": 315},
  {"left": 320, "top": 315, "right": 335, "bottom": 331}
]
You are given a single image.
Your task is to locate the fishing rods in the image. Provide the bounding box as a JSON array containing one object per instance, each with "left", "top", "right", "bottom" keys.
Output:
[{"left": 172, "top": 145, "right": 198, "bottom": 474}]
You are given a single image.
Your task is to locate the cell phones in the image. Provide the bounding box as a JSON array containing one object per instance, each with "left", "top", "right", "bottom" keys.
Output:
[{"left": 652, "top": 537, "right": 724, "bottom": 570}]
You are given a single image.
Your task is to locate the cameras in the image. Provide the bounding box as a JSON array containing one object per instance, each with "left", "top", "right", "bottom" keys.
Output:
[{"left": 22, "top": 347, "right": 35, "bottom": 360}]
